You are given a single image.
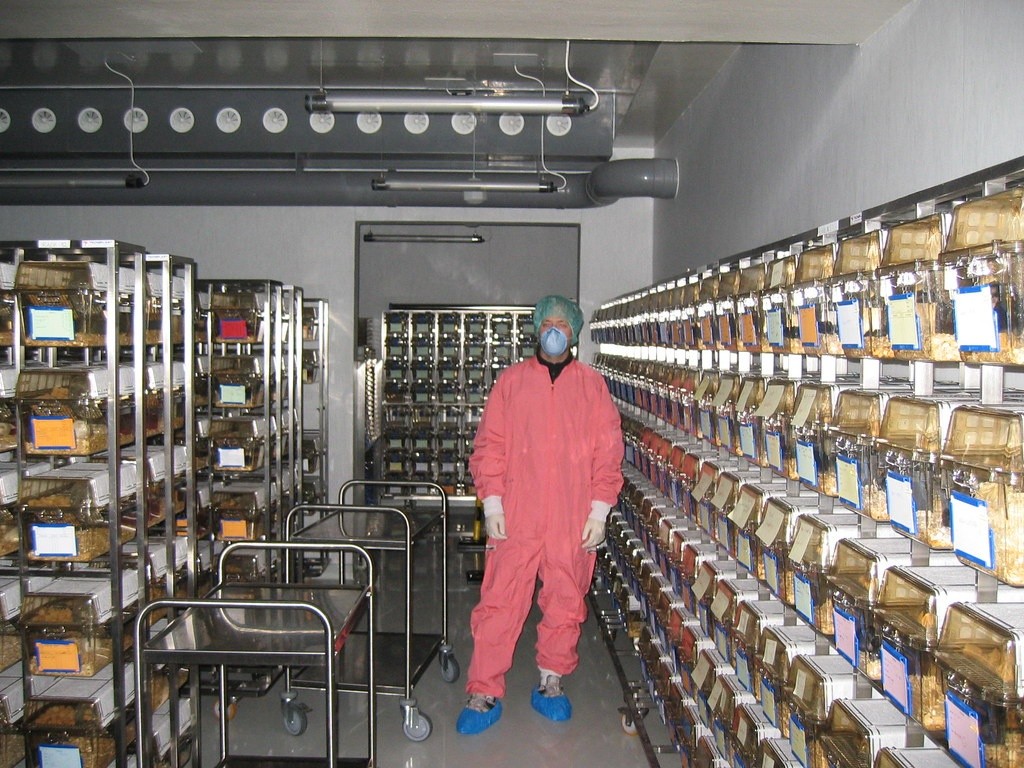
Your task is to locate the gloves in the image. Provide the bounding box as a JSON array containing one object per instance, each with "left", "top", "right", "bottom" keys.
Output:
[
  {"left": 486, "top": 515, "right": 508, "bottom": 540},
  {"left": 581, "top": 518, "right": 606, "bottom": 549}
]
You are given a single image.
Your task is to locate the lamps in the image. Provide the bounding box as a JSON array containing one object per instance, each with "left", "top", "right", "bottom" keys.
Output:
[
  {"left": 304, "top": 37, "right": 601, "bottom": 116},
  {"left": 0, "top": 59, "right": 152, "bottom": 189},
  {"left": 370, "top": 63, "right": 568, "bottom": 194}
]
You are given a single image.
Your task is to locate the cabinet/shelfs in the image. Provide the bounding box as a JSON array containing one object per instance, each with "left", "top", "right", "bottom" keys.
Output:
[
  {"left": 280, "top": 478, "right": 460, "bottom": 740},
  {"left": 196, "top": 278, "right": 286, "bottom": 720},
  {"left": 128, "top": 541, "right": 377, "bottom": 768},
  {"left": 198, "top": 284, "right": 304, "bottom": 582},
  {"left": 381, "top": 307, "right": 534, "bottom": 502},
  {"left": 120, "top": 252, "right": 204, "bottom": 768},
  {"left": 0, "top": 239, "right": 147, "bottom": 768},
  {"left": 588, "top": 156, "right": 1024, "bottom": 768},
  {"left": 215, "top": 296, "right": 330, "bottom": 573}
]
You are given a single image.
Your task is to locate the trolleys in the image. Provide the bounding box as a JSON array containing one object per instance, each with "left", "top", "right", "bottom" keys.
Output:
[
  {"left": 280, "top": 479, "right": 460, "bottom": 742},
  {"left": 133, "top": 540, "right": 377, "bottom": 768}
]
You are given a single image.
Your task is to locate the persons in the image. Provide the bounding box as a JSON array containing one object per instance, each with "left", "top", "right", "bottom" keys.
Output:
[{"left": 452, "top": 292, "right": 625, "bottom": 738}]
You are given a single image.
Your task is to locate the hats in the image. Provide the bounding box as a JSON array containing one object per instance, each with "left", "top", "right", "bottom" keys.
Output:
[{"left": 533, "top": 296, "right": 583, "bottom": 344}]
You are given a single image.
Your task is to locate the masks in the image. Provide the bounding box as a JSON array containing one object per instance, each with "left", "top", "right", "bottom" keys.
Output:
[{"left": 540, "top": 327, "right": 567, "bottom": 356}]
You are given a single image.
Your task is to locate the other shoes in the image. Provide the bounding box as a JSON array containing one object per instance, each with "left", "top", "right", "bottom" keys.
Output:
[
  {"left": 456, "top": 697, "right": 502, "bottom": 733},
  {"left": 532, "top": 686, "right": 571, "bottom": 722}
]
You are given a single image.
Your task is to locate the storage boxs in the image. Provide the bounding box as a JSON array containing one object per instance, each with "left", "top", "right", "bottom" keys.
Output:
[
  {"left": 590, "top": 184, "right": 1024, "bottom": 768},
  {"left": 385, "top": 310, "right": 534, "bottom": 495},
  {"left": 0, "top": 258, "right": 318, "bottom": 768}
]
